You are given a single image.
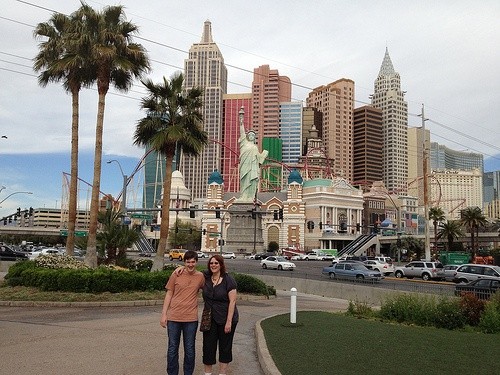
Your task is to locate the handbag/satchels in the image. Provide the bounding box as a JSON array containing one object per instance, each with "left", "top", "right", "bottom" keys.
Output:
[{"left": 199, "top": 309, "right": 212, "bottom": 332}]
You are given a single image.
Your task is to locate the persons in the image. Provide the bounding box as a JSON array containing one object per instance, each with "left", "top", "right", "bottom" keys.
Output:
[
  {"left": 176, "top": 255, "right": 239, "bottom": 375},
  {"left": 238, "top": 121, "right": 268, "bottom": 200},
  {"left": 160, "top": 250, "right": 205, "bottom": 375}
]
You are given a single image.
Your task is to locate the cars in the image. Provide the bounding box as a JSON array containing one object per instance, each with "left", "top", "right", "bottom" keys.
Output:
[
  {"left": 290, "top": 253, "right": 336, "bottom": 262},
  {"left": 255, "top": 252, "right": 275, "bottom": 259},
  {"left": 443, "top": 264, "right": 462, "bottom": 281},
  {"left": 0, "top": 241, "right": 87, "bottom": 260},
  {"left": 262, "top": 256, "right": 296, "bottom": 271},
  {"left": 222, "top": 252, "right": 237, "bottom": 260},
  {"left": 346, "top": 257, "right": 394, "bottom": 277},
  {"left": 322, "top": 263, "right": 385, "bottom": 283},
  {"left": 454, "top": 277, "right": 500, "bottom": 299},
  {"left": 197, "top": 252, "right": 209, "bottom": 258}
]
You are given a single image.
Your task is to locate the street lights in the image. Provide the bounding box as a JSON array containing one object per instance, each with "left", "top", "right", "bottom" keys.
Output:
[{"left": 107, "top": 160, "right": 127, "bottom": 231}]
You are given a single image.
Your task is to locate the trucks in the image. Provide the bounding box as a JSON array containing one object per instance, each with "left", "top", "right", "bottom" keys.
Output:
[{"left": 312, "top": 249, "right": 338, "bottom": 260}]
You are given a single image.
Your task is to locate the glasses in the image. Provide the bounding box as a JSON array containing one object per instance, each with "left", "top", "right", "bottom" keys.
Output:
[{"left": 210, "top": 262, "right": 218, "bottom": 265}]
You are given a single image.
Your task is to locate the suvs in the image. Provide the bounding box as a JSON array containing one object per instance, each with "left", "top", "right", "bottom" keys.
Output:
[
  {"left": 394, "top": 259, "right": 446, "bottom": 280},
  {"left": 169, "top": 249, "right": 189, "bottom": 261},
  {"left": 453, "top": 263, "right": 500, "bottom": 284}
]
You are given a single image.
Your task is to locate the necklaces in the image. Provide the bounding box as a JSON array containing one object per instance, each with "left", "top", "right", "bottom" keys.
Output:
[{"left": 213, "top": 278, "right": 219, "bottom": 284}]
